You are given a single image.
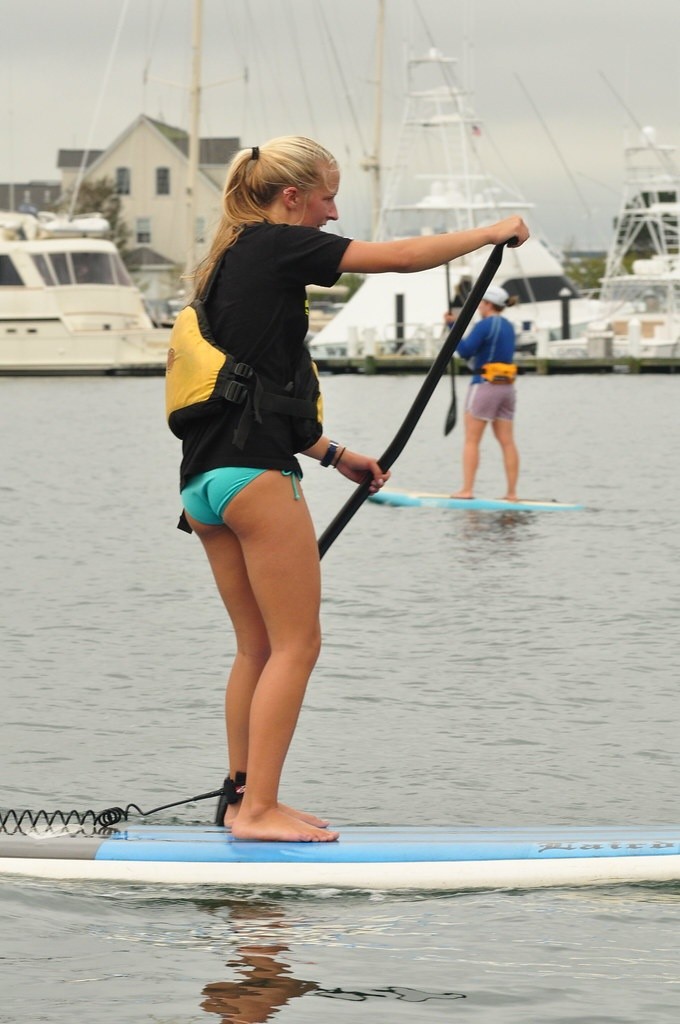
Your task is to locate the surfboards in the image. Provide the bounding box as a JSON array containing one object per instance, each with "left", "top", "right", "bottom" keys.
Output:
[
  {"left": 369, "top": 487, "right": 586, "bottom": 514},
  {"left": 1, "top": 819, "right": 680, "bottom": 889}
]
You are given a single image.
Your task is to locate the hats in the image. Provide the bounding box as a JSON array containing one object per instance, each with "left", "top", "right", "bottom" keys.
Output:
[{"left": 483, "top": 285, "right": 508, "bottom": 307}]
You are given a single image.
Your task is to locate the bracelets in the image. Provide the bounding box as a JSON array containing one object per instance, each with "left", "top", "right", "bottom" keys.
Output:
[
  {"left": 321, "top": 440, "right": 339, "bottom": 468},
  {"left": 334, "top": 447, "right": 346, "bottom": 468}
]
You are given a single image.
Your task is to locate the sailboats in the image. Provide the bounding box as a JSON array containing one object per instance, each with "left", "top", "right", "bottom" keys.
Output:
[{"left": 306, "top": 1, "right": 680, "bottom": 375}]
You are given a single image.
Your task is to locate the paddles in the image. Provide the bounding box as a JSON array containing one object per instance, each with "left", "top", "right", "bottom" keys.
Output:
[
  {"left": 316, "top": 241, "right": 507, "bottom": 561},
  {"left": 442, "top": 261, "right": 458, "bottom": 437}
]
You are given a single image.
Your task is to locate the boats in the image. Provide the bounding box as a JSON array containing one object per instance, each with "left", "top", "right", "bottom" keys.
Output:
[{"left": 0, "top": 238, "right": 175, "bottom": 373}]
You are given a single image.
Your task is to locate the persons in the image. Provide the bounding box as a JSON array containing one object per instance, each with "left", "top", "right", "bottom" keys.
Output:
[
  {"left": 444, "top": 286, "right": 519, "bottom": 500},
  {"left": 167, "top": 133, "right": 532, "bottom": 844}
]
